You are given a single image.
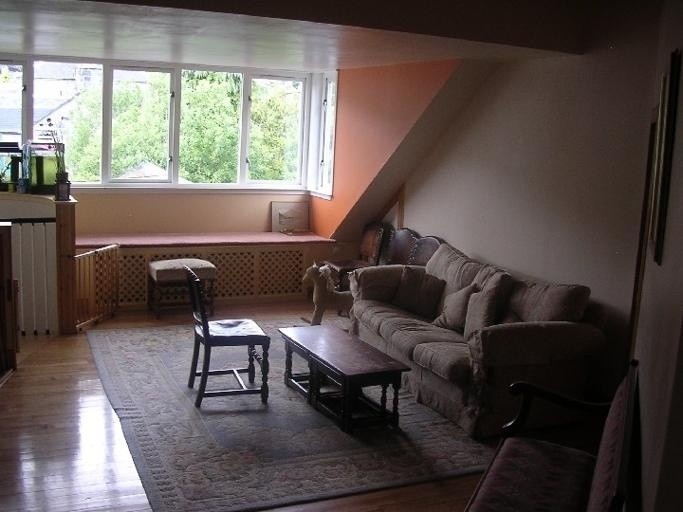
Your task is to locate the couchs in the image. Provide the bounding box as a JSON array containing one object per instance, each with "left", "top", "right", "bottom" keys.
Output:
[{"left": 347, "top": 242, "right": 618, "bottom": 442}]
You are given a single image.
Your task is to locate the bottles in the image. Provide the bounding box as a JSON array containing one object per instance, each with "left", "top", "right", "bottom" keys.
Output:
[{"left": 7, "top": 182, "right": 14, "bottom": 193}]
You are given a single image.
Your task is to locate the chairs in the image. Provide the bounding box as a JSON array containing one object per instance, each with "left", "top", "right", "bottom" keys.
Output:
[
  {"left": 322, "top": 221, "right": 385, "bottom": 316},
  {"left": 413, "top": 235, "right": 441, "bottom": 265},
  {"left": 462, "top": 358, "right": 638, "bottom": 511},
  {"left": 378, "top": 227, "right": 417, "bottom": 266},
  {"left": 183, "top": 264, "right": 270, "bottom": 410}
]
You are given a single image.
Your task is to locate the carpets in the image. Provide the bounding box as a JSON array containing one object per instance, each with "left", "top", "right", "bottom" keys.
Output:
[{"left": 84, "top": 314, "right": 497, "bottom": 511}]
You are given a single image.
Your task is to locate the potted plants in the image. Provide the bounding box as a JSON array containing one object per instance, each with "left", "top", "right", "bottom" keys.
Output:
[{"left": 38, "top": 117, "right": 67, "bottom": 182}]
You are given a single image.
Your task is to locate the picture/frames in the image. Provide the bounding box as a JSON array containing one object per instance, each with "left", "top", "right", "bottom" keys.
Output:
[
  {"left": 647, "top": 49, "right": 683, "bottom": 264},
  {"left": 271, "top": 201, "right": 309, "bottom": 233}
]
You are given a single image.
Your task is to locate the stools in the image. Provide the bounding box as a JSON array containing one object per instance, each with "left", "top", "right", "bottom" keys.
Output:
[{"left": 147, "top": 258, "right": 217, "bottom": 320}]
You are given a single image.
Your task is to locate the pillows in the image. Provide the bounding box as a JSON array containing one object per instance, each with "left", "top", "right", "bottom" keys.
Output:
[
  {"left": 498, "top": 310, "right": 523, "bottom": 324},
  {"left": 464, "top": 286, "right": 496, "bottom": 341},
  {"left": 431, "top": 282, "right": 477, "bottom": 329},
  {"left": 392, "top": 265, "right": 447, "bottom": 318},
  {"left": 472, "top": 263, "right": 513, "bottom": 321}
]
